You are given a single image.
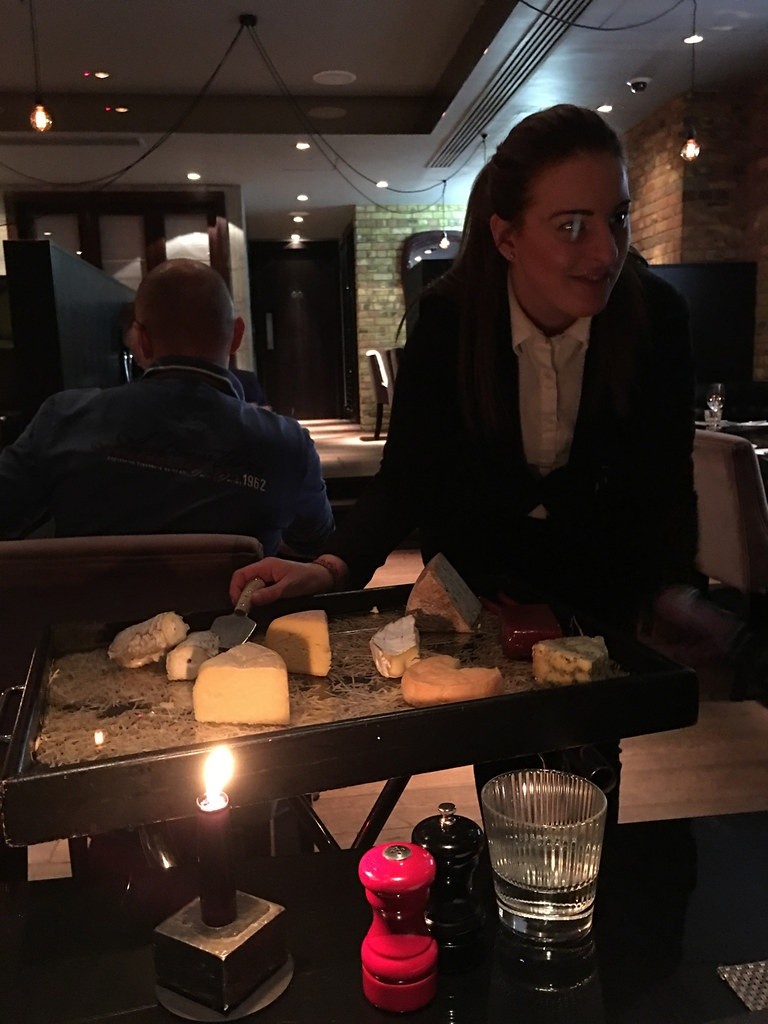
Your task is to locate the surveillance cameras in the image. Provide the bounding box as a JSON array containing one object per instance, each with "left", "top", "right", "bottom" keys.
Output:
[{"left": 627, "top": 76, "right": 651, "bottom": 94}]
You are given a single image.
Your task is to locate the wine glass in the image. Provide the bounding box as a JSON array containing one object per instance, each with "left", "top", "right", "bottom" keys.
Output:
[{"left": 704, "top": 382, "right": 725, "bottom": 431}]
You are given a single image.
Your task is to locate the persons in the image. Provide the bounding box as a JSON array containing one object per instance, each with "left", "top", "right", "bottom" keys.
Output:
[
  {"left": 230, "top": 104, "right": 732, "bottom": 826},
  {"left": 0, "top": 259, "right": 336, "bottom": 563}
]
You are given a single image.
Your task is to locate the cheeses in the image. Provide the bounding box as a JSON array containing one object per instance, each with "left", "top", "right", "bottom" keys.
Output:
[{"left": 108, "top": 552, "right": 609, "bottom": 725}]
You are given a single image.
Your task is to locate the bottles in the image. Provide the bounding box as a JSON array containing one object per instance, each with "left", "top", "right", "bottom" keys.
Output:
[
  {"left": 358, "top": 842, "right": 438, "bottom": 1011},
  {"left": 411, "top": 801, "right": 484, "bottom": 947}
]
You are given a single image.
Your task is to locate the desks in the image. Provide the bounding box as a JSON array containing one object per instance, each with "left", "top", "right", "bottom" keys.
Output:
[
  {"left": 694, "top": 425, "right": 768, "bottom": 475},
  {"left": 0, "top": 809, "right": 768, "bottom": 1023}
]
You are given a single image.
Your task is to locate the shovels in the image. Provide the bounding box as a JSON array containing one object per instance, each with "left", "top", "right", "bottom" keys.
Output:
[{"left": 206, "top": 576, "right": 266, "bottom": 650}]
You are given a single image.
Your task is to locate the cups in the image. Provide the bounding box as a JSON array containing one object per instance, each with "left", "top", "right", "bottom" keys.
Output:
[{"left": 479, "top": 769, "right": 607, "bottom": 940}]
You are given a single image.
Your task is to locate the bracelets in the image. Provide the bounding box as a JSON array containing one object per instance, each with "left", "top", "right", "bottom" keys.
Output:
[{"left": 312, "top": 559, "right": 338, "bottom": 585}]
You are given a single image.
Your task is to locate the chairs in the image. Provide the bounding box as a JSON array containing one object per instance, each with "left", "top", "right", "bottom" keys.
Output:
[
  {"left": 380, "top": 347, "right": 404, "bottom": 409},
  {"left": 364, "top": 349, "right": 389, "bottom": 440},
  {"left": 0, "top": 534, "right": 276, "bottom": 878},
  {"left": 692, "top": 430, "right": 768, "bottom": 701}
]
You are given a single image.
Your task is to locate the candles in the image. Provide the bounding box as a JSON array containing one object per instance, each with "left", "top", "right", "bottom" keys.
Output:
[{"left": 193, "top": 743, "right": 237, "bottom": 927}]
